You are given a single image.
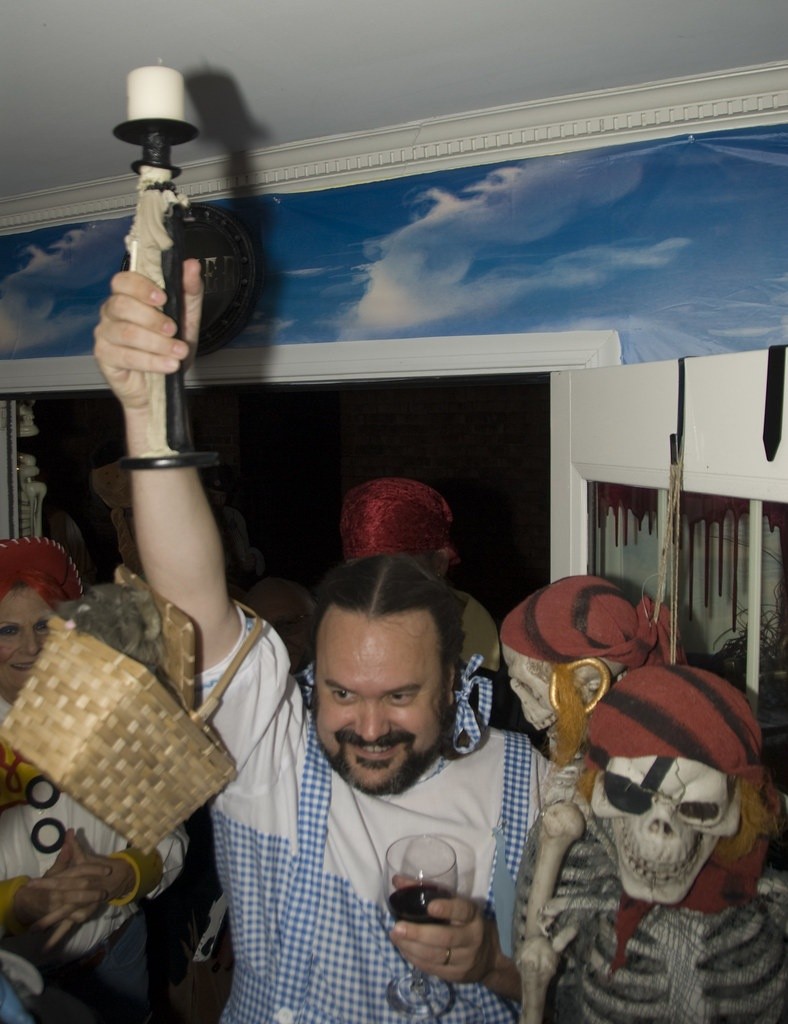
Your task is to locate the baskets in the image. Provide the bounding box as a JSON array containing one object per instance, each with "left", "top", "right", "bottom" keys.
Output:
[{"left": 0, "top": 597, "right": 263, "bottom": 856}]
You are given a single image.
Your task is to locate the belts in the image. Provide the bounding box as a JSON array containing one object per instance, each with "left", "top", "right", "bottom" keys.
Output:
[{"left": 68, "top": 916, "right": 134, "bottom": 978}]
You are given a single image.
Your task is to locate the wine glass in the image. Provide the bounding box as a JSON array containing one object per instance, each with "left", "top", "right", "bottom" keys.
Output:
[{"left": 384, "top": 834, "right": 459, "bottom": 1017}]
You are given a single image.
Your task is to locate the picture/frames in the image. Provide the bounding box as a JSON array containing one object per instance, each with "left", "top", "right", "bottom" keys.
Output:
[{"left": 117, "top": 204, "right": 263, "bottom": 360}]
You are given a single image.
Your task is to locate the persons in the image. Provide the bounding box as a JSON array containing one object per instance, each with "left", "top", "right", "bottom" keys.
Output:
[
  {"left": 92, "top": 261, "right": 554, "bottom": 1024},
  {"left": 0, "top": 537, "right": 189, "bottom": 1024}
]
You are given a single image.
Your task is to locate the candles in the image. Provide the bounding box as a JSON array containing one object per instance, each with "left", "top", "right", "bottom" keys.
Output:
[{"left": 126, "top": 59, "right": 185, "bottom": 119}]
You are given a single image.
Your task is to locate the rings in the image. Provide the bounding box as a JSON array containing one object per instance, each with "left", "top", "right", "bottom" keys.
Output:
[{"left": 444, "top": 948, "right": 451, "bottom": 966}]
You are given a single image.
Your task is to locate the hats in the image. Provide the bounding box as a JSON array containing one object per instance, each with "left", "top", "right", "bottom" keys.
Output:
[{"left": 0, "top": 536, "right": 83, "bottom": 601}]
